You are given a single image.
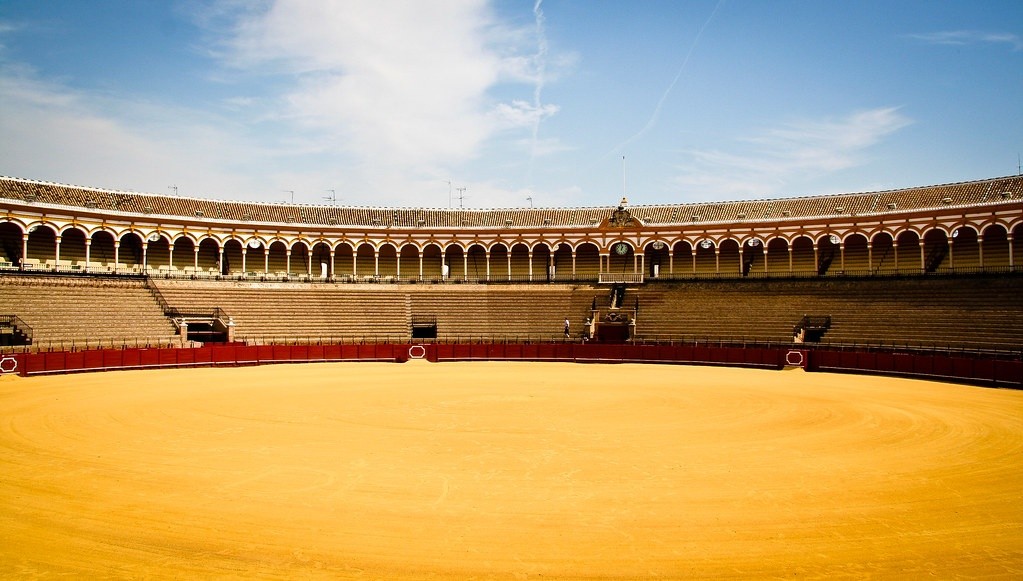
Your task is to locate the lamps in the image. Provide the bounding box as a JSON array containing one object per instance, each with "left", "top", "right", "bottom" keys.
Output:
[
  {"left": 643, "top": 217, "right": 652, "bottom": 223},
  {"left": 419, "top": 220, "right": 425, "bottom": 226},
  {"left": 738, "top": 214, "right": 746, "bottom": 220},
  {"left": 506, "top": 220, "right": 512, "bottom": 226},
  {"left": 942, "top": 198, "right": 953, "bottom": 206},
  {"left": 836, "top": 208, "right": 844, "bottom": 215},
  {"left": 887, "top": 203, "right": 897, "bottom": 211},
  {"left": 589, "top": 219, "right": 597, "bottom": 224},
  {"left": 782, "top": 211, "right": 791, "bottom": 218},
  {"left": 544, "top": 219, "right": 551, "bottom": 225},
  {"left": 692, "top": 216, "right": 700, "bottom": 222},
  {"left": 1002, "top": 192, "right": 1012, "bottom": 200}
]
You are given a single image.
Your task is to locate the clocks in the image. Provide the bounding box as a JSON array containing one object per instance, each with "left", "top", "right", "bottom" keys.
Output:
[{"left": 616, "top": 244, "right": 628, "bottom": 255}]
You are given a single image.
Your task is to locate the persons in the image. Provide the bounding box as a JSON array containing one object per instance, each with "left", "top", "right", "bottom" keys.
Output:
[{"left": 564, "top": 317, "right": 570, "bottom": 337}]
